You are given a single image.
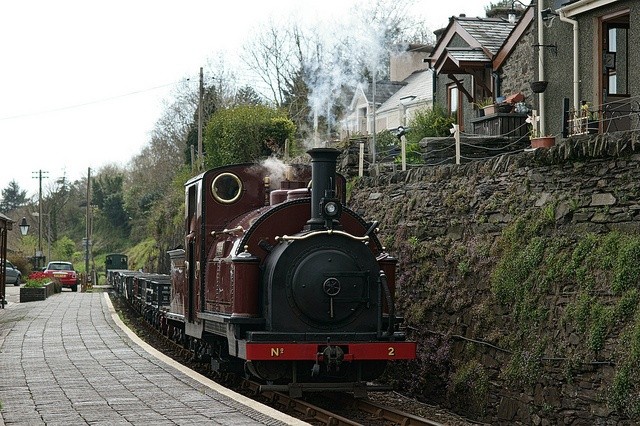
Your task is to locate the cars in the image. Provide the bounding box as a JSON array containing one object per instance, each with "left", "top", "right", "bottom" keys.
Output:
[
  {"left": 44, "top": 259, "right": 77, "bottom": 291},
  {"left": 1, "top": 259, "right": 22, "bottom": 285}
]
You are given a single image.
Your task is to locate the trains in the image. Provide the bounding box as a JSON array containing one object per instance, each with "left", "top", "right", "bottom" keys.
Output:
[{"left": 105, "top": 147, "right": 416, "bottom": 405}]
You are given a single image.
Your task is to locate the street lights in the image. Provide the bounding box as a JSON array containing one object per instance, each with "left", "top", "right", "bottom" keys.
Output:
[{"left": 33, "top": 211, "right": 51, "bottom": 262}]
